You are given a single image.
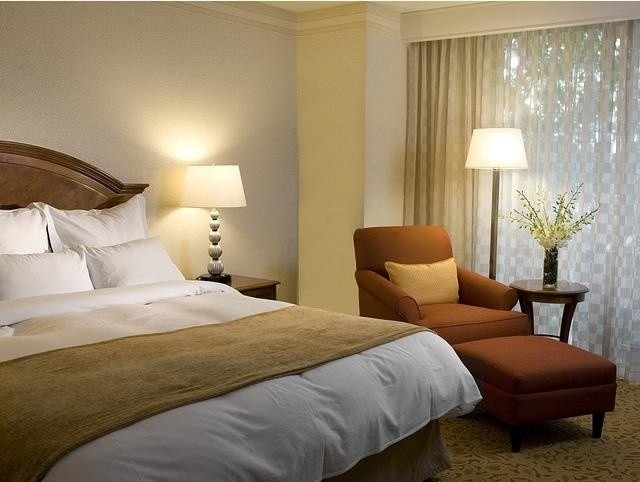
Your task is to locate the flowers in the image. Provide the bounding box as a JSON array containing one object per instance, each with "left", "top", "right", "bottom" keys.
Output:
[{"left": 496, "top": 183, "right": 600, "bottom": 285}]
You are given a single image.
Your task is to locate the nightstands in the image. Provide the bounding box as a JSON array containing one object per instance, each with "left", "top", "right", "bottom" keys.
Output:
[{"left": 231, "top": 274, "right": 281, "bottom": 300}]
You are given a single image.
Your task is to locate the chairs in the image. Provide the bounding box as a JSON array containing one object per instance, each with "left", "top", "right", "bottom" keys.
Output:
[{"left": 353, "top": 225, "right": 529, "bottom": 370}]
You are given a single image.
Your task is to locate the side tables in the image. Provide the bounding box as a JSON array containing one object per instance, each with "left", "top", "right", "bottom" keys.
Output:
[{"left": 509, "top": 279, "right": 590, "bottom": 344}]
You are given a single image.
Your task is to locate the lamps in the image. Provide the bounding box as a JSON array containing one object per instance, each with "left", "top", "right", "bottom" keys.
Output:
[
  {"left": 465, "top": 127, "right": 528, "bottom": 281},
  {"left": 179, "top": 164, "right": 247, "bottom": 284}
]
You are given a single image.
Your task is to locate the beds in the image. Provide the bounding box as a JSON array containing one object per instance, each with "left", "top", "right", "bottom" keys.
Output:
[{"left": 0, "top": 139, "right": 483, "bottom": 482}]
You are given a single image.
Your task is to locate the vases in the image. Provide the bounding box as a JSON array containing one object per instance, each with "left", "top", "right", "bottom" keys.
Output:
[{"left": 542, "top": 251, "right": 557, "bottom": 289}]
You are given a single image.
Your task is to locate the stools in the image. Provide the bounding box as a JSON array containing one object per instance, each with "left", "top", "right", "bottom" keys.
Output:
[{"left": 453, "top": 335, "right": 617, "bottom": 453}]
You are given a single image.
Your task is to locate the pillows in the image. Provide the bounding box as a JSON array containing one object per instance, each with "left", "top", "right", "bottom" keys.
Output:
[
  {"left": 385, "top": 257, "right": 460, "bottom": 304},
  {"left": 31, "top": 193, "right": 148, "bottom": 252},
  {"left": 0, "top": 204, "right": 50, "bottom": 252},
  {"left": 0, "top": 245, "right": 93, "bottom": 301},
  {"left": 82, "top": 235, "right": 185, "bottom": 289}
]
